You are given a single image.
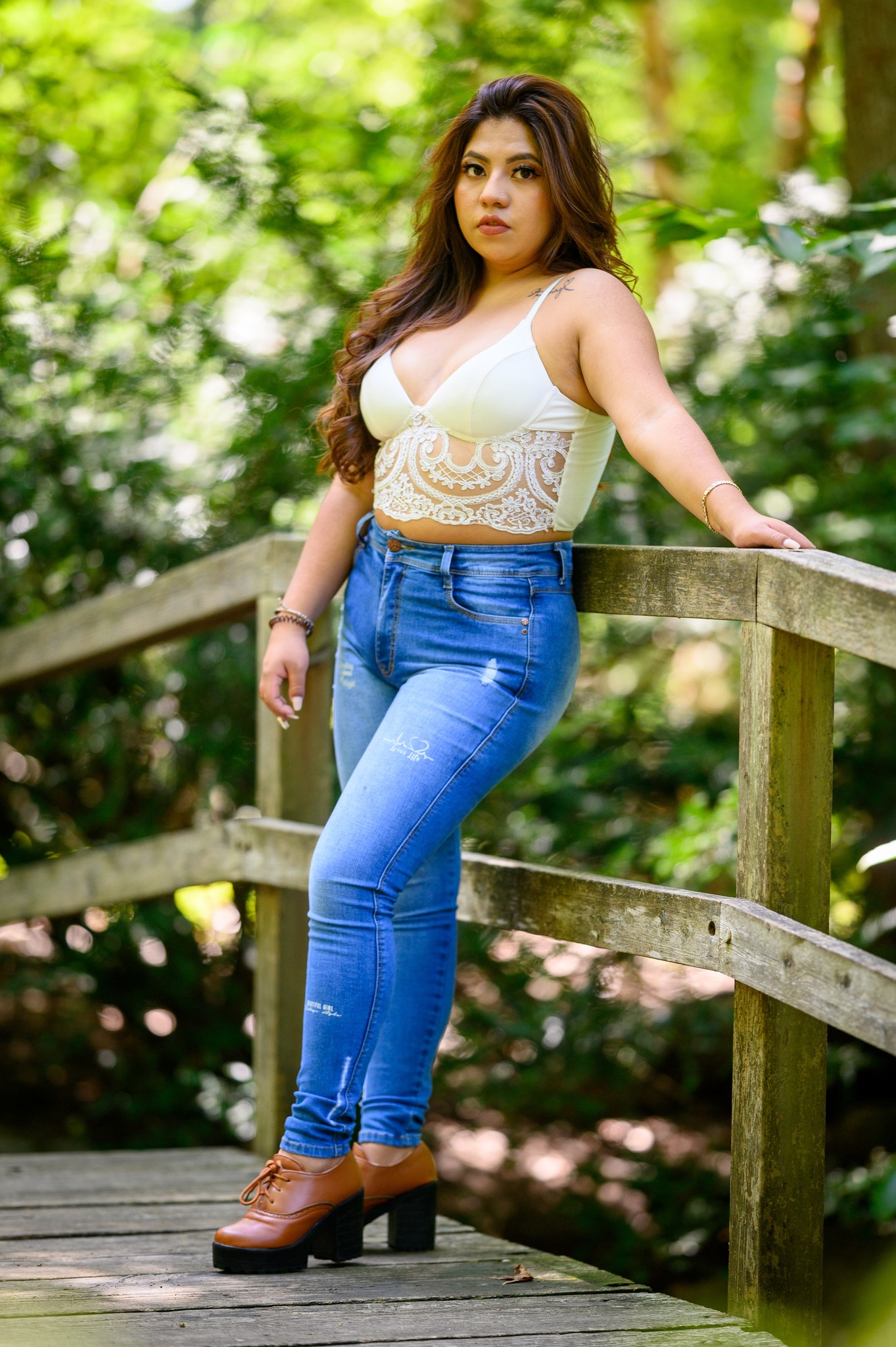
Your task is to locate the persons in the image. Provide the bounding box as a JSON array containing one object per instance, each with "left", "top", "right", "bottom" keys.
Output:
[{"left": 213, "top": 73, "right": 817, "bottom": 1278}]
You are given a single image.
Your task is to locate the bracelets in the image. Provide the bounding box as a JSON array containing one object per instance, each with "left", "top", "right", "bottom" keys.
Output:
[
  {"left": 270, "top": 598, "right": 314, "bottom": 639},
  {"left": 702, "top": 481, "right": 743, "bottom": 534}
]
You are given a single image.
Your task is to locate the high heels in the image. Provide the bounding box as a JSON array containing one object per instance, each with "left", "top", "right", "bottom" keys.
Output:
[
  {"left": 313, "top": 1140, "right": 441, "bottom": 1258},
  {"left": 212, "top": 1151, "right": 365, "bottom": 1276}
]
